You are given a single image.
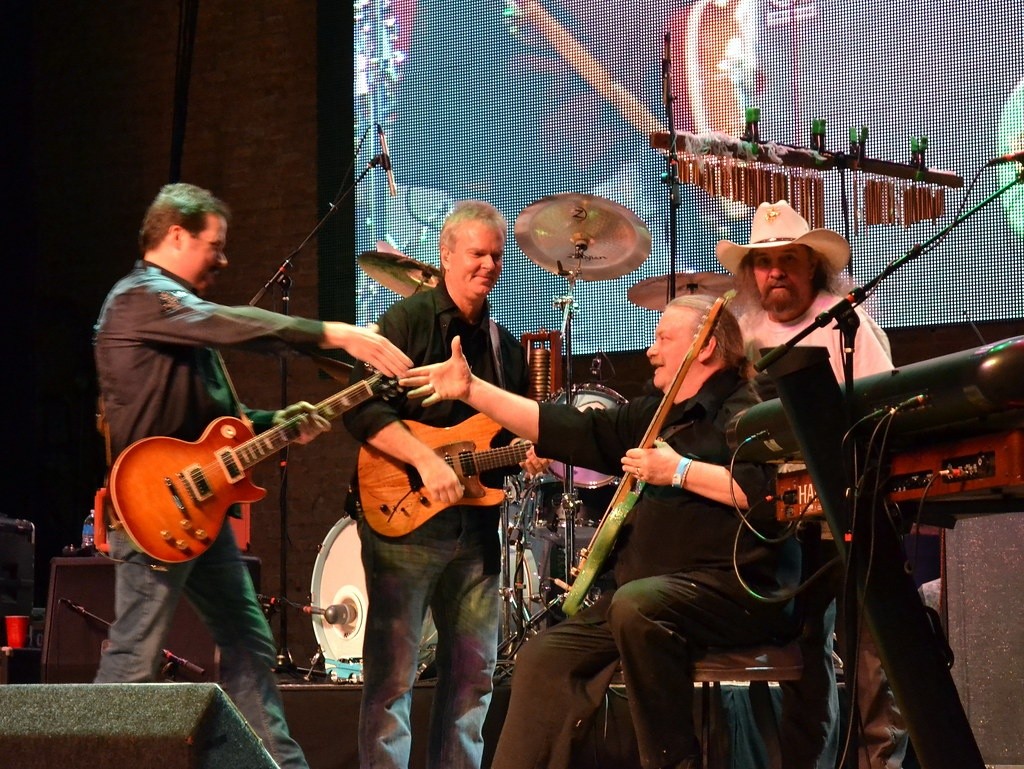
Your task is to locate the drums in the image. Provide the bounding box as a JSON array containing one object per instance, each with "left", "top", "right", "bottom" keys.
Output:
[
  {"left": 309, "top": 513, "right": 541, "bottom": 683},
  {"left": 542, "top": 383, "right": 630, "bottom": 489}
]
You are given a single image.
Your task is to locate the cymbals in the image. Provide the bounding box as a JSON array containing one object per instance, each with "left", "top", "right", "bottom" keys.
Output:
[
  {"left": 623, "top": 274, "right": 737, "bottom": 311},
  {"left": 513, "top": 191, "right": 654, "bottom": 280},
  {"left": 355, "top": 250, "right": 442, "bottom": 299}
]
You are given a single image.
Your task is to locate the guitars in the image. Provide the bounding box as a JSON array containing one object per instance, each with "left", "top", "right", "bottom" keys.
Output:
[
  {"left": 562, "top": 297, "right": 725, "bottom": 615},
  {"left": 356, "top": 389, "right": 538, "bottom": 537},
  {"left": 113, "top": 362, "right": 404, "bottom": 564}
]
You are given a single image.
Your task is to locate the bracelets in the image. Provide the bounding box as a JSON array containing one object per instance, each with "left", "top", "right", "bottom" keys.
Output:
[
  {"left": 671, "top": 457, "right": 689, "bottom": 488},
  {"left": 681, "top": 459, "right": 693, "bottom": 489}
]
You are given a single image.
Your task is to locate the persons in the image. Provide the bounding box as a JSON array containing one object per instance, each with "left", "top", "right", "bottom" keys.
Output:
[
  {"left": 95, "top": 184, "right": 413, "bottom": 769},
  {"left": 340, "top": 200, "right": 553, "bottom": 769},
  {"left": 719, "top": 200, "right": 894, "bottom": 769},
  {"left": 398, "top": 295, "right": 802, "bottom": 769}
]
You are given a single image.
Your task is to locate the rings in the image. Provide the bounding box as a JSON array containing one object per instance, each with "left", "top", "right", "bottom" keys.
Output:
[{"left": 637, "top": 467, "right": 640, "bottom": 475}]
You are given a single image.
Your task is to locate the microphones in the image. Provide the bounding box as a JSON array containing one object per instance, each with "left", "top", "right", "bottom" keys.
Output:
[
  {"left": 324, "top": 604, "right": 356, "bottom": 625},
  {"left": 989, "top": 150, "right": 1024, "bottom": 167},
  {"left": 664, "top": 34, "right": 672, "bottom": 105},
  {"left": 377, "top": 125, "right": 397, "bottom": 196},
  {"left": 60, "top": 597, "right": 80, "bottom": 612}
]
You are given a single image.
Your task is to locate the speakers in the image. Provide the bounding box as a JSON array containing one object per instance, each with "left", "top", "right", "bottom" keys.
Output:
[
  {"left": 940, "top": 511, "right": 1024, "bottom": 769},
  {"left": 1, "top": 520, "right": 283, "bottom": 769}
]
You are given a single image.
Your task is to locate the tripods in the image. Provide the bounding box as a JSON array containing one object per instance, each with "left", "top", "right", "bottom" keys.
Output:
[{"left": 498, "top": 251, "right": 597, "bottom": 655}]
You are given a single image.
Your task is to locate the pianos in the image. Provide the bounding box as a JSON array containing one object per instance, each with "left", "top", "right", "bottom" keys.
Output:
[{"left": 727, "top": 332, "right": 1024, "bottom": 769}]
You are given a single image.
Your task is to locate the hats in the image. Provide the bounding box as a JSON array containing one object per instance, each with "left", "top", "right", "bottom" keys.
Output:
[{"left": 715, "top": 199, "right": 851, "bottom": 279}]
